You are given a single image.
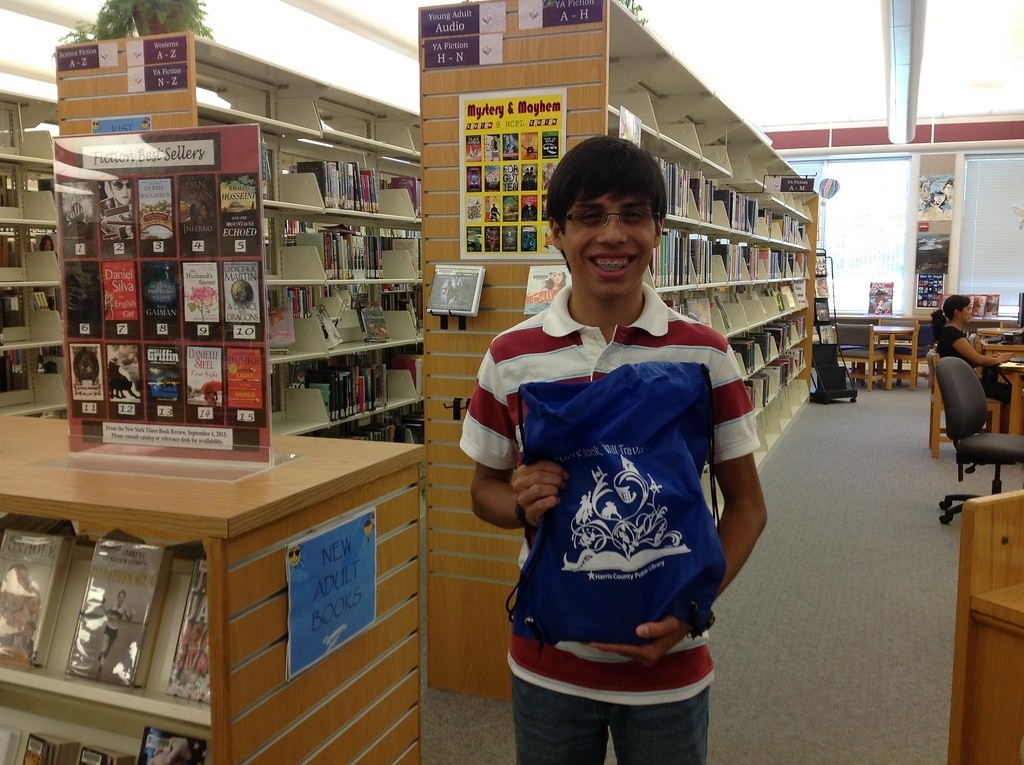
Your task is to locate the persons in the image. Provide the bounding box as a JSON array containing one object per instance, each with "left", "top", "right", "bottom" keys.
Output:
[
  {"left": 460, "top": 134, "right": 770, "bottom": 765},
  {"left": 544, "top": 271, "right": 567, "bottom": 294},
  {"left": 94, "top": 589, "right": 136, "bottom": 669},
  {"left": 931, "top": 295, "right": 1016, "bottom": 405}
]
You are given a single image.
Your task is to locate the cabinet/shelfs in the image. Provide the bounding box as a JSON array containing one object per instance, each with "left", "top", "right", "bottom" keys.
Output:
[
  {"left": 60, "top": 31, "right": 422, "bottom": 444},
  {"left": 0, "top": 409, "right": 424, "bottom": 765},
  {"left": 419, "top": 1, "right": 820, "bottom": 699},
  {"left": 0, "top": 88, "right": 60, "bottom": 417},
  {"left": 947, "top": 489, "right": 1024, "bottom": 765}
]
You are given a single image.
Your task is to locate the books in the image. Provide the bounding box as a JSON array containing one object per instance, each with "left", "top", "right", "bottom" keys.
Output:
[
  {"left": 163, "top": 559, "right": 210, "bottom": 705},
  {"left": 651, "top": 153, "right": 837, "bottom": 410},
  {"left": 868, "top": 283, "right": 894, "bottom": 314},
  {"left": 0, "top": 725, "right": 207, "bottom": 764},
  {"left": 267, "top": 162, "right": 425, "bottom": 445},
  {"left": 65, "top": 537, "right": 176, "bottom": 689},
  {"left": 0, "top": 171, "right": 63, "bottom": 392},
  {"left": 427, "top": 265, "right": 486, "bottom": 318},
  {"left": 968, "top": 294, "right": 1000, "bottom": 319},
  {"left": 58, "top": 174, "right": 264, "bottom": 426},
  {"left": 0, "top": 528, "right": 74, "bottom": 670}
]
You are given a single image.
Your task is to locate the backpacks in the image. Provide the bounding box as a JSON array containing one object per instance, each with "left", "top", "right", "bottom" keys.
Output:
[{"left": 505, "top": 361, "right": 727, "bottom": 647}]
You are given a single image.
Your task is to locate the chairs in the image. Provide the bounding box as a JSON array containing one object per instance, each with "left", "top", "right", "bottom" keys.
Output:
[
  {"left": 937, "top": 356, "right": 1024, "bottom": 526},
  {"left": 833, "top": 324, "right": 885, "bottom": 391},
  {"left": 893, "top": 323, "right": 934, "bottom": 391}
]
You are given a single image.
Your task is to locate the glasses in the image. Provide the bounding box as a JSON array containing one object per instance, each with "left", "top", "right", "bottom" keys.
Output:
[{"left": 557, "top": 205, "right": 661, "bottom": 227}]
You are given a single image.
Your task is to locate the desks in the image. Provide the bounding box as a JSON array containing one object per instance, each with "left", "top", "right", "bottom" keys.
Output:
[
  {"left": 869, "top": 325, "right": 913, "bottom": 391},
  {"left": 992, "top": 352, "right": 1024, "bottom": 435}
]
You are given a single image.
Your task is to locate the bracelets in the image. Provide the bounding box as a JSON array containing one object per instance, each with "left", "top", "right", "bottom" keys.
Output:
[{"left": 515, "top": 498, "right": 539, "bottom": 535}]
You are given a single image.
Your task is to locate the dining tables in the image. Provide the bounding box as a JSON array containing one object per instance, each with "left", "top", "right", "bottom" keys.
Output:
[{"left": 925, "top": 350, "right": 1000, "bottom": 460}]
